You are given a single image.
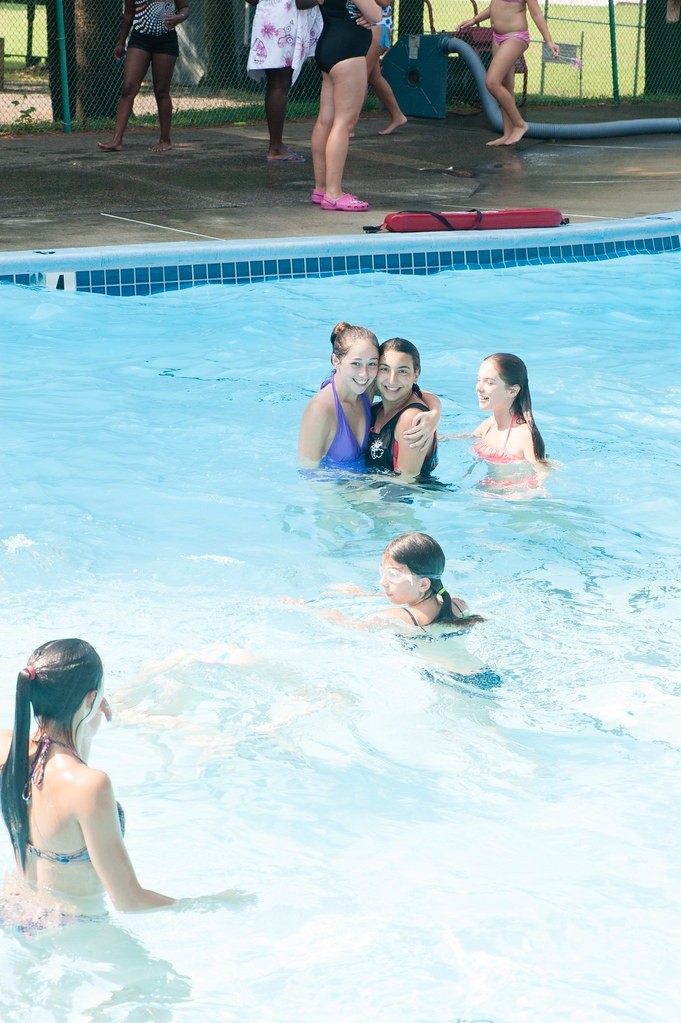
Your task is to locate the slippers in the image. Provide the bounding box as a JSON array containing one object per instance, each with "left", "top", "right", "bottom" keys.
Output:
[{"left": 266, "top": 147, "right": 306, "bottom": 163}]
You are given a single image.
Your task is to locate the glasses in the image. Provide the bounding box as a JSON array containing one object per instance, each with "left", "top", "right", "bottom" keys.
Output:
[{"left": 380, "top": 565, "right": 426, "bottom": 582}]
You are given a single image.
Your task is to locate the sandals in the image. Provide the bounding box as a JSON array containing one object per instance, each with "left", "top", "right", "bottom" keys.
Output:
[
  {"left": 321, "top": 193, "right": 370, "bottom": 211},
  {"left": 311, "top": 189, "right": 358, "bottom": 204}
]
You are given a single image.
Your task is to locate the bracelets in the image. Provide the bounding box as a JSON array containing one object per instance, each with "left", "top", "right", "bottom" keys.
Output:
[
  {"left": 180, "top": 14, "right": 185, "bottom": 18},
  {"left": 475, "top": 18, "right": 477, "bottom": 24}
]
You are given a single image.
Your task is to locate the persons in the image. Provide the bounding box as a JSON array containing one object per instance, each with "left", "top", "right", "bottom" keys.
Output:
[
  {"left": 456, "top": 0, "right": 561, "bottom": 146},
  {"left": 372, "top": 530, "right": 469, "bottom": 626},
  {"left": 311, "top": 0, "right": 407, "bottom": 213},
  {"left": 95, "top": 0, "right": 191, "bottom": 152},
  {"left": 246, "top": 0, "right": 325, "bottom": 165},
  {"left": 363, "top": 338, "right": 437, "bottom": 478},
  {"left": 474, "top": 353, "right": 547, "bottom": 462},
  {"left": 0, "top": 637, "right": 176, "bottom": 913},
  {"left": 300, "top": 324, "right": 443, "bottom": 470}
]
